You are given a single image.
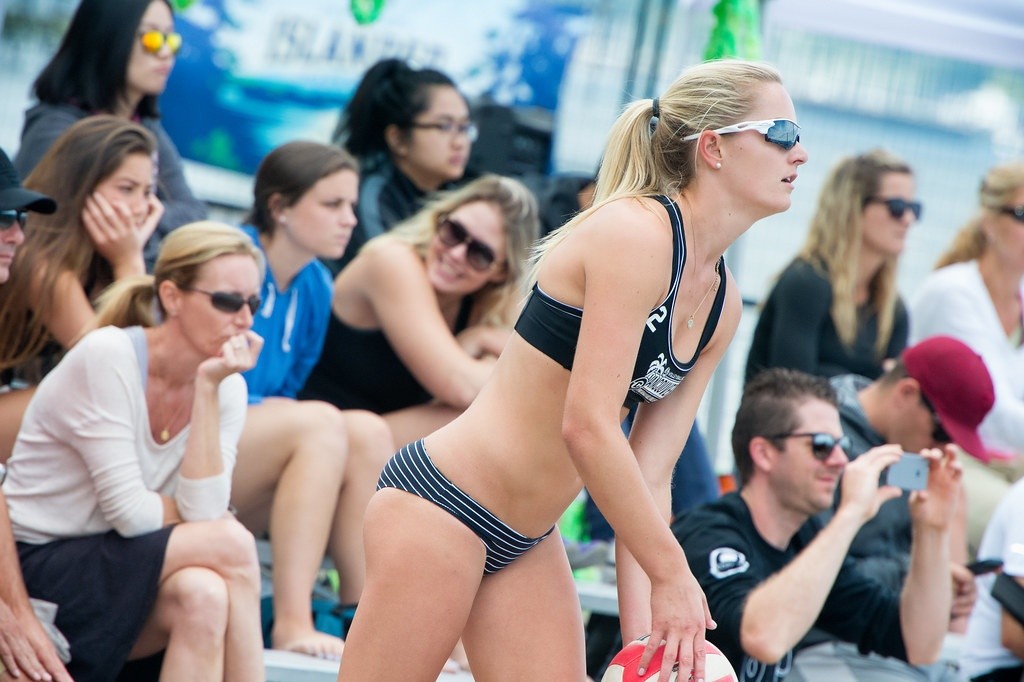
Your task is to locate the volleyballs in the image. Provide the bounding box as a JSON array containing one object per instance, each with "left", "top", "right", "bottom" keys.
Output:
[{"left": 600, "top": 632, "right": 742, "bottom": 682}]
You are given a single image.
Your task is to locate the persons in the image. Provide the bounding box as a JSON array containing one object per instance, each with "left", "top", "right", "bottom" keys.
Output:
[
  {"left": 339, "top": 59, "right": 807, "bottom": 682},
  {"left": 295, "top": 174, "right": 540, "bottom": 461},
  {"left": 907, "top": 165, "right": 1024, "bottom": 682},
  {"left": 319, "top": 53, "right": 479, "bottom": 289},
  {"left": 745, "top": 148, "right": 924, "bottom": 399},
  {"left": 665, "top": 368, "right": 963, "bottom": 682},
  {"left": 0, "top": 1, "right": 394, "bottom": 682},
  {"left": 803, "top": 336, "right": 1013, "bottom": 682}
]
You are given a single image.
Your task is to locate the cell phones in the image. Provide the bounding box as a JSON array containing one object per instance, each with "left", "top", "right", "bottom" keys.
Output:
[{"left": 885, "top": 453, "right": 928, "bottom": 491}]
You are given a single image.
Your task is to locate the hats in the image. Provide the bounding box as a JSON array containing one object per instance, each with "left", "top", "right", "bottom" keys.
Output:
[
  {"left": 0, "top": 145, "right": 57, "bottom": 215},
  {"left": 900, "top": 334, "right": 996, "bottom": 465}
]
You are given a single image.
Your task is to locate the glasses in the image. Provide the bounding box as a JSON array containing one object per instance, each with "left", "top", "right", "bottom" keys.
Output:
[
  {"left": 177, "top": 286, "right": 261, "bottom": 316},
  {"left": 681, "top": 119, "right": 803, "bottom": 150},
  {"left": 863, "top": 196, "right": 922, "bottom": 221},
  {"left": 0, "top": 210, "right": 27, "bottom": 230},
  {"left": 903, "top": 374, "right": 953, "bottom": 444},
  {"left": 997, "top": 205, "right": 1024, "bottom": 223},
  {"left": 136, "top": 29, "right": 182, "bottom": 53},
  {"left": 410, "top": 118, "right": 480, "bottom": 142},
  {"left": 437, "top": 213, "right": 500, "bottom": 274},
  {"left": 764, "top": 432, "right": 853, "bottom": 464}
]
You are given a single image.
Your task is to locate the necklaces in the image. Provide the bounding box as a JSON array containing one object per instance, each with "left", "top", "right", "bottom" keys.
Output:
[
  {"left": 149, "top": 327, "right": 197, "bottom": 442},
  {"left": 683, "top": 203, "right": 717, "bottom": 330}
]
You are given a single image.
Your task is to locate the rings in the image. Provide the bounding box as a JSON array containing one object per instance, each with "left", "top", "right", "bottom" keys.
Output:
[{"left": 234, "top": 341, "right": 244, "bottom": 353}]
